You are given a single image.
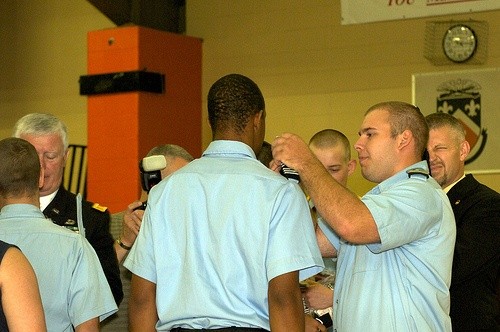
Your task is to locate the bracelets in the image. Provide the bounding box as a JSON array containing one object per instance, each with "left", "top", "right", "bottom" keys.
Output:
[
  {"left": 115, "top": 238, "right": 131, "bottom": 251},
  {"left": 327, "top": 283, "right": 334, "bottom": 292}
]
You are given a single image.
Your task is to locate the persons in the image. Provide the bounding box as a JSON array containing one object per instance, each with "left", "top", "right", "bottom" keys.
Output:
[
  {"left": 11, "top": 112, "right": 124, "bottom": 326},
  {"left": 270, "top": 101, "right": 459, "bottom": 332},
  {"left": 0, "top": 240, "right": 49, "bottom": 332},
  {"left": 421, "top": 112, "right": 500, "bottom": 331},
  {"left": 1, "top": 137, "right": 120, "bottom": 332},
  {"left": 99, "top": 144, "right": 194, "bottom": 332},
  {"left": 122, "top": 73, "right": 326, "bottom": 332},
  {"left": 299, "top": 128, "right": 362, "bottom": 331}
]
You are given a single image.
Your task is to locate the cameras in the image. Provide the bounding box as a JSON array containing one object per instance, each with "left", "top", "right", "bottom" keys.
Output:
[{"left": 132, "top": 155, "right": 166, "bottom": 212}]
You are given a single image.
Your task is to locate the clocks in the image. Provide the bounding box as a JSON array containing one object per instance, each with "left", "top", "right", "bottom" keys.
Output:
[{"left": 441, "top": 24, "right": 479, "bottom": 64}]
